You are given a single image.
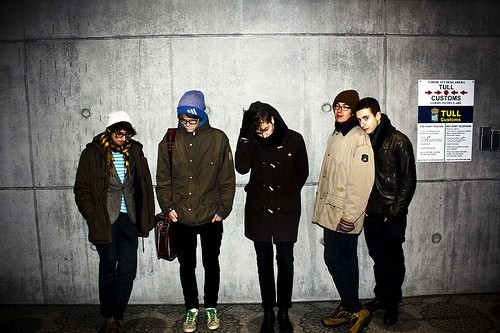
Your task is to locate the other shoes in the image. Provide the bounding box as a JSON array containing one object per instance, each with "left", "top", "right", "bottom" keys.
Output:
[
  {"left": 103, "top": 317, "right": 118, "bottom": 333},
  {"left": 277, "top": 312, "right": 294, "bottom": 333},
  {"left": 382, "top": 305, "right": 399, "bottom": 326},
  {"left": 259, "top": 309, "right": 276, "bottom": 333},
  {"left": 363, "top": 297, "right": 380, "bottom": 313}
]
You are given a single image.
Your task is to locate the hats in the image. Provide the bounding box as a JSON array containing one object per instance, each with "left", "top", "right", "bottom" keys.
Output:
[
  {"left": 177, "top": 90, "right": 205, "bottom": 119},
  {"left": 333, "top": 89, "right": 360, "bottom": 113},
  {"left": 105, "top": 111, "right": 134, "bottom": 129}
]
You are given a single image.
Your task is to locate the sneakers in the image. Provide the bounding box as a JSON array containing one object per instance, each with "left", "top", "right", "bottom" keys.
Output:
[
  {"left": 205, "top": 307, "right": 220, "bottom": 330},
  {"left": 183, "top": 307, "right": 198, "bottom": 332},
  {"left": 320, "top": 304, "right": 362, "bottom": 327},
  {"left": 349, "top": 309, "right": 373, "bottom": 333}
]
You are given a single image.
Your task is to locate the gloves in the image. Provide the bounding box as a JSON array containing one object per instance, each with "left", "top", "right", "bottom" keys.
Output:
[
  {"left": 239, "top": 109, "right": 253, "bottom": 141},
  {"left": 335, "top": 219, "right": 355, "bottom": 233}
]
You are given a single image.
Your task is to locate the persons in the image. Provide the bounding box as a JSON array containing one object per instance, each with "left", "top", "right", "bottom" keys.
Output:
[
  {"left": 312, "top": 90, "right": 373, "bottom": 333},
  {"left": 235, "top": 101, "right": 309, "bottom": 333},
  {"left": 73, "top": 112, "right": 155, "bottom": 333},
  {"left": 354, "top": 98, "right": 416, "bottom": 325},
  {"left": 156, "top": 91, "right": 236, "bottom": 333}
]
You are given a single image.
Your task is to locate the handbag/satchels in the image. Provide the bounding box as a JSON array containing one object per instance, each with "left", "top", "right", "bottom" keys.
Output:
[{"left": 154, "top": 212, "right": 178, "bottom": 262}]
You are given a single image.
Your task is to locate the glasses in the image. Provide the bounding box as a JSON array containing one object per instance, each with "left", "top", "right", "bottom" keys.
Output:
[
  {"left": 333, "top": 104, "right": 351, "bottom": 111},
  {"left": 179, "top": 118, "right": 198, "bottom": 125},
  {"left": 111, "top": 130, "right": 133, "bottom": 138}
]
